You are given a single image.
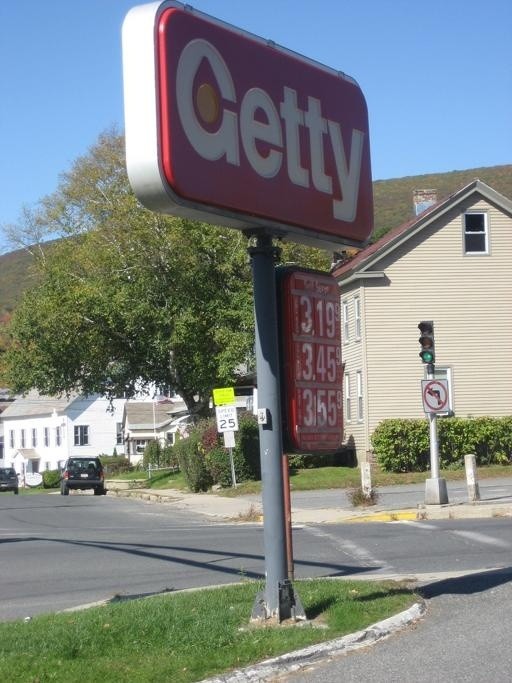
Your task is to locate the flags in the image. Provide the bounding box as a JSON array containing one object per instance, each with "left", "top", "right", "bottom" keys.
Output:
[{"left": 157, "top": 395, "right": 175, "bottom": 404}]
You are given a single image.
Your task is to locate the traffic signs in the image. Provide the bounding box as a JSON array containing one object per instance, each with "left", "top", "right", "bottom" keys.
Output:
[{"left": 216, "top": 405, "right": 238, "bottom": 432}]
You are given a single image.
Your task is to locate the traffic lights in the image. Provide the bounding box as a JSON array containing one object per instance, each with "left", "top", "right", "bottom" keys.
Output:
[{"left": 418, "top": 321, "right": 436, "bottom": 365}]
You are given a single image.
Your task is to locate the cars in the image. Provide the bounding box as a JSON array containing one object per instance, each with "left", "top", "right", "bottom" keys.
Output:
[
  {"left": 61, "top": 457, "right": 104, "bottom": 495},
  {"left": 0, "top": 467, "right": 20, "bottom": 494}
]
